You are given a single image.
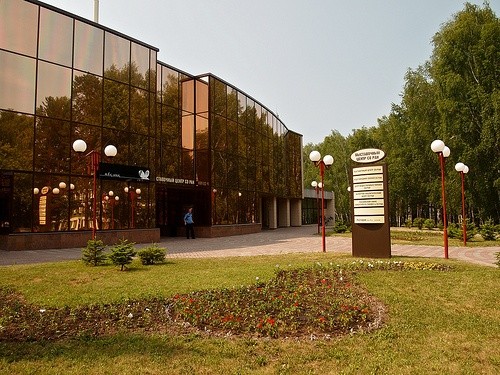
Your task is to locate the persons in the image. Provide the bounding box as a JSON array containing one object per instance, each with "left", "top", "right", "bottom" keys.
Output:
[{"left": 184, "top": 208, "right": 195, "bottom": 239}]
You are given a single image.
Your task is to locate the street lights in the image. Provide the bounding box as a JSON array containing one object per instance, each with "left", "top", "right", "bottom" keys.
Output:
[
  {"left": 73, "top": 139, "right": 117, "bottom": 265},
  {"left": 33, "top": 182, "right": 142, "bottom": 231},
  {"left": 454, "top": 162, "right": 469, "bottom": 247},
  {"left": 310, "top": 180, "right": 325, "bottom": 234},
  {"left": 308, "top": 149, "right": 334, "bottom": 252},
  {"left": 430, "top": 139, "right": 451, "bottom": 259}
]
